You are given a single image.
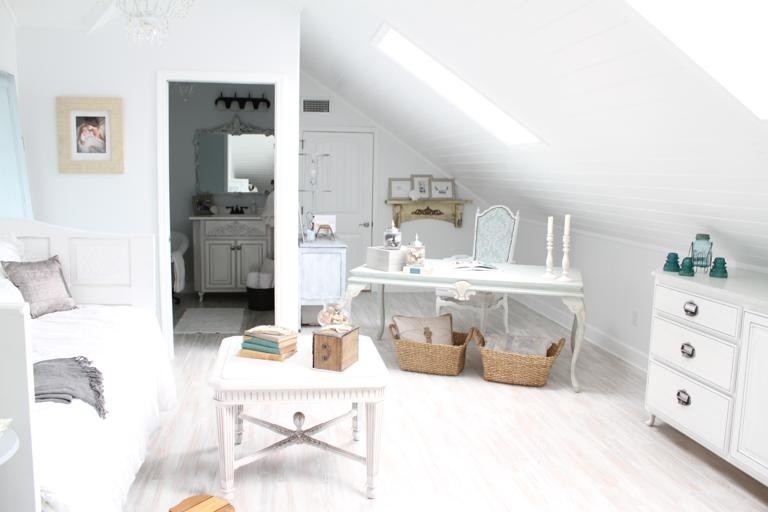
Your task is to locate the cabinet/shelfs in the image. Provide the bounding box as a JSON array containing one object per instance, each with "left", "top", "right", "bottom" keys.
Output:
[
  {"left": 297, "top": 231, "right": 348, "bottom": 329},
  {"left": 189, "top": 212, "right": 273, "bottom": 304},
  {"left": 645, "top": 264, "right": 768, "bottom": 493}
]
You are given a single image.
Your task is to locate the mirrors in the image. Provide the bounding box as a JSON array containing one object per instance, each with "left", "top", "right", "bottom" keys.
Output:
[{"left": 193, "top": 115, "right": 274, "bottom": 208}]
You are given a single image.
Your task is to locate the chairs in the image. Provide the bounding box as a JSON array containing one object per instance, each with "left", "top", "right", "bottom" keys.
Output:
[{"left": 434, "top": 202, "right": 520, "bottom": 340}]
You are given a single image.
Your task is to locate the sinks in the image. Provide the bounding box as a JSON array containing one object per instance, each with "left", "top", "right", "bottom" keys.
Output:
[{"left": 172, "top": 231, "right": 188, "bottom": 262}]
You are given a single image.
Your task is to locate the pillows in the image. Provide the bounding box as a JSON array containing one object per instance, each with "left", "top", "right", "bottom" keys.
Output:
[
  {"left": 483, "top": 332, "right": 548, "bottom": 357},
  {"left": 0, "top": 256, "right": 76, "bottom": 320},
  {"left": 391, "top": 314, "right": 454, "bottom": 346}
]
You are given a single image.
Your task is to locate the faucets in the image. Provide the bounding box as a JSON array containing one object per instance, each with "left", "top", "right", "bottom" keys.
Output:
[{"left": 225, "top": 204, "right": 248, "bottom": 214}]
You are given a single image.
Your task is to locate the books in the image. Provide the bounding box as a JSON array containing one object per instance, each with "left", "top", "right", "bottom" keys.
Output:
[{"left": 239, "top": 324, "right": 298, "bottom": 361}]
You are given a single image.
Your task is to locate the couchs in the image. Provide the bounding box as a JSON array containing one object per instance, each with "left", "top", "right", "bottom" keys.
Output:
[{"left": 0, "top": 219, "right": 151, "bottom": 512}]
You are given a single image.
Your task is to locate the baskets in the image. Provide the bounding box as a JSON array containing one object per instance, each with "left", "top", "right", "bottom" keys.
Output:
[
  {"left": 389, "top": 324, "right": 475, "bottom": 377},
  {"left": 474, "top": 328, "right": 565, "bottom": 389}
]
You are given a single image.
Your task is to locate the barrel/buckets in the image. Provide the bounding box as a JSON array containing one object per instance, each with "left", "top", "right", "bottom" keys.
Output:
[{"left": 247, "top": 287, "right": 275, "bottom": 310}]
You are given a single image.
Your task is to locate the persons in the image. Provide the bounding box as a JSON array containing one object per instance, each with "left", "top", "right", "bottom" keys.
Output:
[
  {"left": 80, "top": 124, "right": 98, "bottom": 152},
  {"left": 85, "top": 116, "right": 105, "bottom": 143}
]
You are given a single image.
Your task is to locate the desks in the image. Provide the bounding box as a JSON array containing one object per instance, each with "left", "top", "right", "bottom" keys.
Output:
[{"left": 348, "top": 260, "right": 587, "bottom": 391}]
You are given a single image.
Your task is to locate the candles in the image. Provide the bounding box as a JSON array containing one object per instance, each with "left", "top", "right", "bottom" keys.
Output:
[
  {"left": 561, "top": 214, "right": 572, "bottom": 282},
  {"left": 546, "top": 215, "right": 554, "bottom": 280}
]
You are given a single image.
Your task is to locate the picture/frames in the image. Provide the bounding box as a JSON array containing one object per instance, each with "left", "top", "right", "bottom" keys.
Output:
[
  {"left": 411, "top": 175, "right": 431, "bottom": 199},
  {"left": 193, "top": 193, "right": 214, "bottom": 216},
  {"left": 430, "top": 179, "right": 456, "bottom": 201},
  {"left": 57, "top": 96, "right": 124, "bottom": 174},
  {"left": 388, "top": 178, "right": 411, "bottom": 199}
]
